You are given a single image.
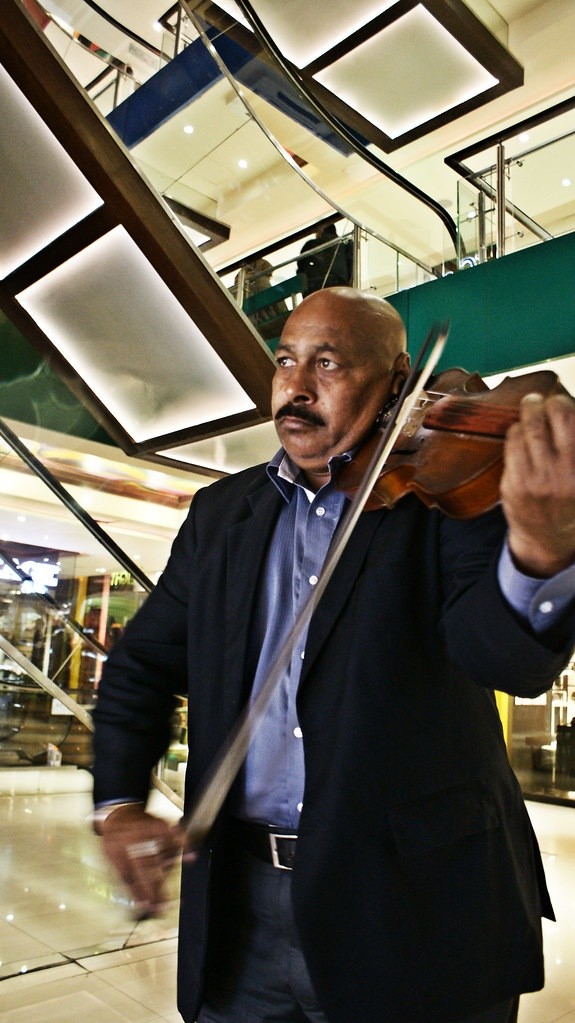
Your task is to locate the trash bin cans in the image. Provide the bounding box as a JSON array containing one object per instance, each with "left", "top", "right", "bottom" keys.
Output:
[{"left": 555, "top": 725, "right": 575, "bottom": 791}]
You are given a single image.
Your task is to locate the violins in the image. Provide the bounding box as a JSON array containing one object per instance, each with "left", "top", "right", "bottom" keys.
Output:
[{"left": 334, "top": 368, "right": 575, "bottom": 522}]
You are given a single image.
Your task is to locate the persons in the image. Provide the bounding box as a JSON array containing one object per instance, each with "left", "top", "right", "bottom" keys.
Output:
[
  {"left": 296, "top": 223, "right": 354, "bottom": 300},
  {"left": 92, "top": 286, "right": 575, "bottom": 1023},
  {"left": 227, "top": 259, "right": 290, "bottom": 342},
  {"left": 31, "top": 615, "right": 70, "bottom": 687}
]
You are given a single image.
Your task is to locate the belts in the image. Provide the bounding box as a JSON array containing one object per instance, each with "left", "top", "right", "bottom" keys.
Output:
[{"left": 236, "top": 820, "right": 299, "bottom": 869}]
